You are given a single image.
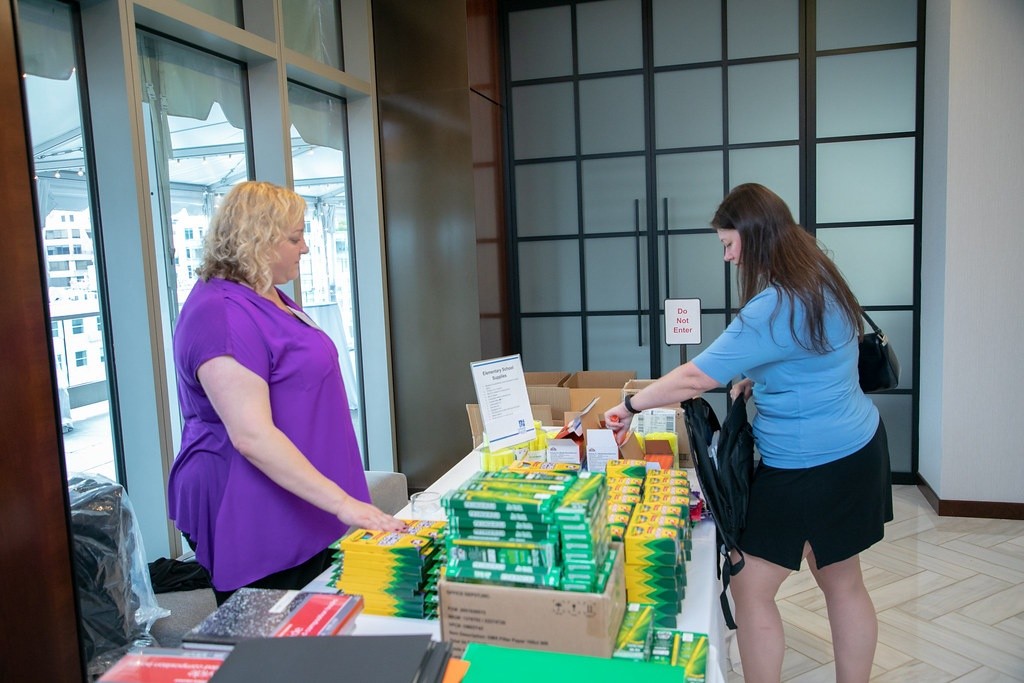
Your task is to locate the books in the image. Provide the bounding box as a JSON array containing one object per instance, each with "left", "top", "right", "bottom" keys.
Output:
[{"left": 94, "top": 587, "right": 365, "bottom": 683}]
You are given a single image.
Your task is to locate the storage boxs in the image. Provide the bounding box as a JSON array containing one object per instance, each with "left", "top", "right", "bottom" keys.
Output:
[
  {"left": 465, "top": 370, "right": 698, "bottom": 472},
  {"left": 437, "top": 543, "right": 627, "bottom": 661}
]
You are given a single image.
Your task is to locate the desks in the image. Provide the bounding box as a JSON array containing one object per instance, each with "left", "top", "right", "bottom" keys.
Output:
[{"left": 302, "top": 426, "right": 733, "bottom": 683}]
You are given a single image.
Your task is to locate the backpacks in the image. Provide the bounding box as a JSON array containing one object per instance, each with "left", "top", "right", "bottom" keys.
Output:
[{"left": 682, "top": 392, "right": 757, "bottom": 630}]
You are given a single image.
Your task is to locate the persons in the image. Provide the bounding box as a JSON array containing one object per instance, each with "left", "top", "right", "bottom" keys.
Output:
[
  {"left": 167, "top": 181, "right": 408, "bottom": 608},
  {"left": 604, "top": 183, "right": 894, "bottom": 683}
]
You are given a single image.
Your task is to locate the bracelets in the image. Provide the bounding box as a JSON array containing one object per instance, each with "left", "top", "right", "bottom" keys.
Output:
[{"left": 625, "top": 394, "right": 641, "bottom": 414}]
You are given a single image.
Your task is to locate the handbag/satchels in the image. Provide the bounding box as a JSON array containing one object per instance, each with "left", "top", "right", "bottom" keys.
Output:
[{"left": 858, "top": 306, "right": 899, "bottom": 394}]
